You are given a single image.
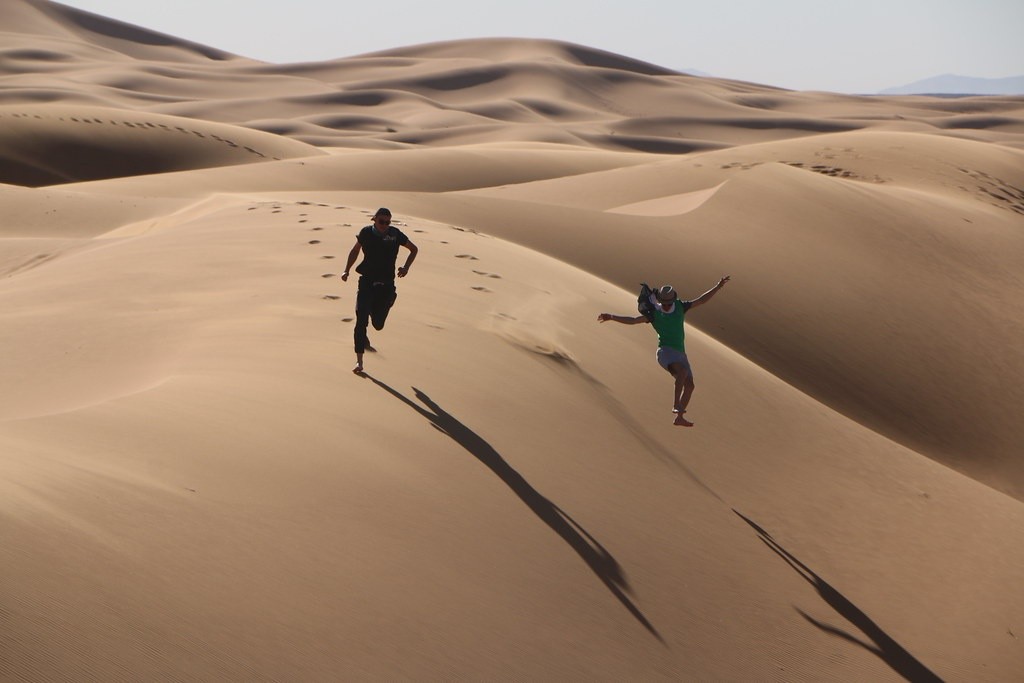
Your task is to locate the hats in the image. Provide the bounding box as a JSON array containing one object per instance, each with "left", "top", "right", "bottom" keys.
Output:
[{"left": 657, "top": 286, "right": 677, "bottom": 305}]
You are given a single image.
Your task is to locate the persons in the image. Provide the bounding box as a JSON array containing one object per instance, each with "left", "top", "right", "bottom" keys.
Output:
[
  {"left": 340, "top": 207, "right": 418, "bottom": 372},
  {"left": 597, "top": 274, "right": 731, "bottom": 426}
]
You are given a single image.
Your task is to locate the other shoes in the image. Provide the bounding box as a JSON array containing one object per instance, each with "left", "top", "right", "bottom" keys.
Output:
[
  {"left": 672, "top": 404, "right": 686, "bottom": 413},
  {"left": 353, "top": 363, "right": 363, "bottom": 373},
  {"left": 673, "top": 417, "right": 694, "bottom": 427}
]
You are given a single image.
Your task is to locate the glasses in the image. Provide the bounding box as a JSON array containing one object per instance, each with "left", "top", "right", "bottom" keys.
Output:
[{"left": 379, "top": 219, "right": 391, "bottom": 225}]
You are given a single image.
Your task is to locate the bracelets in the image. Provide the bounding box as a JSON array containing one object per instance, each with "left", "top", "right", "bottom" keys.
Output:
[
  {"left": 611, "top": 315, "right": 614, "bottom": 320},
  {"left": 717, "top": 282, "right": 723, "bottom": 287}
]
